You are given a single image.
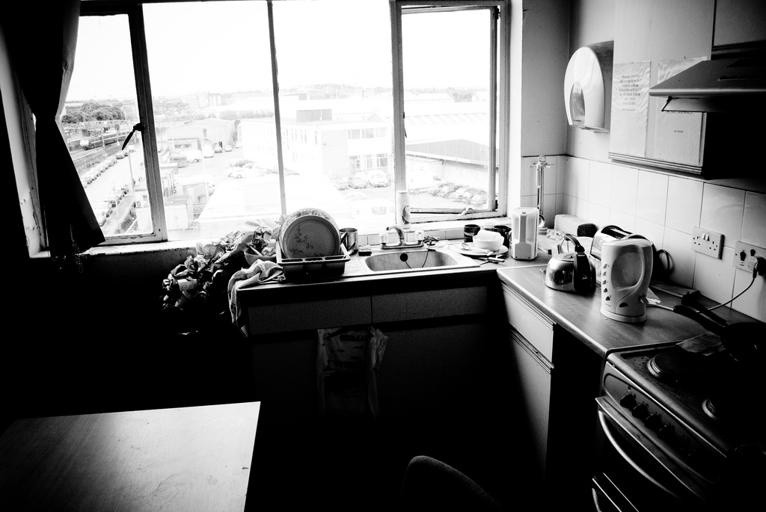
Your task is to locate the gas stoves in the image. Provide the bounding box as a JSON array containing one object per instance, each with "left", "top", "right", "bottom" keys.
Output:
[{"left": 600, "top": 336, "right": 766, "bottom": 507}]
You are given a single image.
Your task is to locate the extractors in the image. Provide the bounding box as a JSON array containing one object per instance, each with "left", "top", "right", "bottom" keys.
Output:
[{"left": 649, "top": 0, "right": 766, "bottom": 120}]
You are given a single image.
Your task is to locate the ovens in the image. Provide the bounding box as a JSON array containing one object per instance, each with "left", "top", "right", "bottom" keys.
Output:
[{"left": 585, "top": 397, "right": 726, "bottom": 512}]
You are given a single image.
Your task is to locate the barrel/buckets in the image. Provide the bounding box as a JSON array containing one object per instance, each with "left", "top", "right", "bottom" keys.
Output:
[{"left": 278, "top": 210, "right": 341, "bottom": 260}]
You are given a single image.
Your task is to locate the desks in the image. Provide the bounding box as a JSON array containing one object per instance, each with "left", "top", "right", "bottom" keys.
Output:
[{"left": 1, "top": 396, "right": 266, "bottom": 512}]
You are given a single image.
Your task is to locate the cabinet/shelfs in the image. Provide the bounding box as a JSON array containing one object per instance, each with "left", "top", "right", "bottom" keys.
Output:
[
  {"left": 606, "top": 0, "right": 766, "bottom": 183},
  {"left": 373, "top": 280, "right": 494, "bottom": 419},
  {"left": 494, "top": 271, "right": 604, "bottom": 486},
  {"left": 235, "top": 283, "right": 372, "bottom": 417}
]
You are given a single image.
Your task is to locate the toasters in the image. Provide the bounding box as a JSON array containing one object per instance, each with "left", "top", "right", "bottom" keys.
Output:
[{"left": 592, "top": 225, "right": 649, "bottom": 264}]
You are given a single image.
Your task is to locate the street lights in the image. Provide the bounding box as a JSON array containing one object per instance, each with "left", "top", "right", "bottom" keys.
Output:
[{"left": 125, "top": 146, "right": 136, "bottom": 187}]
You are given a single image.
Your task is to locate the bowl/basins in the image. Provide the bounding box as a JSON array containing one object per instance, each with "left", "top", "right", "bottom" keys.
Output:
[{"left": 241, "top": 249, "right": 275, "bottom": 265}]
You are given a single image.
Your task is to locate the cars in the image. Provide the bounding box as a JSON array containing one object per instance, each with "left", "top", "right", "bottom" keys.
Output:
[
  {"left": 333, "top": 171, "right": 487, "bottom": 207},
  {"left": 96, "top": 177, "right": 143, "bottom": 229},
  {"left": 82, "top": 147, "right": 133, "bottom": 189},
  {"left": 177, "top": 139, "right": 241, "bottom": 165}
]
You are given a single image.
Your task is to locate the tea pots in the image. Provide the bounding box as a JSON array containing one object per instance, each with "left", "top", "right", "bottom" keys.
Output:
[{"left": 545, "top": 233, "right": 596, "bottom": 295}]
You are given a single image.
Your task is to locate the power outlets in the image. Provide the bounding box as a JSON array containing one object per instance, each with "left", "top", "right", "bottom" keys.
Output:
[
  {"left": 733, "top": 241, "right": 765, "bottom": 279},
  {"left": 686, "top": 225, "right": 725, "bottom": 261}
]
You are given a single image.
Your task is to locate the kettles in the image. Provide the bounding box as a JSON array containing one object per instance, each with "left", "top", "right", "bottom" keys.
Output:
[{"left": 600, "top": 239, "right": 654, "bottom": 324}]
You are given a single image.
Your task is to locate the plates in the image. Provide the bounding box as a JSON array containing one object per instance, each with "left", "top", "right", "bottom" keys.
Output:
[
  {"left": 279, "top": 211, "right": 340, "bottom": 258},
  {"left": 460, "top": 243, "right": 509, "bottom": 257}
]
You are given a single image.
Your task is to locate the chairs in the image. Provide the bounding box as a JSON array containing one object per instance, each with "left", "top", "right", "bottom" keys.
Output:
[{"left": 371, "top": 455, "right": 498, "bottom": 512}]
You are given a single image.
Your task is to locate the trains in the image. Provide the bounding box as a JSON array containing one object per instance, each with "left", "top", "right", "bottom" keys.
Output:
[{"left": 80, "top": 129, "right": 131, "bottom": 151}]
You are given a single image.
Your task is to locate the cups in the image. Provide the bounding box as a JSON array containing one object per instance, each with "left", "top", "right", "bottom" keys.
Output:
[
  {"left": 494, "top": 224, "right": 510, "bottom": 248},
  {"left": 509, "top": 207, "right": 540, "bottom": 260},
  {"left": 338, "top": 227, "right": 358, "bottom": 254},
  {"left": 463, "top": 224, "right": 480, "bottom": 243},
  {"left": 472, "top": 235, "right": 504, "bottom": 252}
]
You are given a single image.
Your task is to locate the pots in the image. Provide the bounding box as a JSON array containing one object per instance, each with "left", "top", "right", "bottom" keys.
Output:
[{"left": 671, "top": 293, "right": 766, "bottom": 374}]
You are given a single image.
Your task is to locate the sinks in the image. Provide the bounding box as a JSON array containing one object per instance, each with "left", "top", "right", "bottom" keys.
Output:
[{"left": 365, "top": 246, "right": 480, "bottom": 273}]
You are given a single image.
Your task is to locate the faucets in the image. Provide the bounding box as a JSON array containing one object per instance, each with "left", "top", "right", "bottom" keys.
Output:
[{"left": 385, "top": 226, "right": 407, "bottom": 246}]
